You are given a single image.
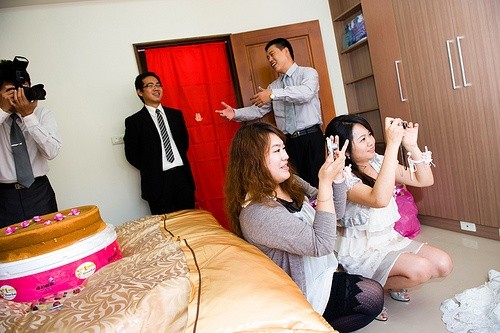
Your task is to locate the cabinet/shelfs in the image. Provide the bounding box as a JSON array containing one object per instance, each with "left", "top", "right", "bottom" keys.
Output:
[{"left": 327, "top": 0, "right": 500, "bottom": 241}]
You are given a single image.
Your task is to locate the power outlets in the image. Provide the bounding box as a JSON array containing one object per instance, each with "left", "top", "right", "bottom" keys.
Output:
[{"left": 460, "top": 220, "right": 477, "bottom": 232}]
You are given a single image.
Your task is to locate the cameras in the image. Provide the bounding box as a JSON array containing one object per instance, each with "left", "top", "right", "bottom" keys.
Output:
[
  {"left": 5, "top": 55, "right": 52, "bottom": 104},
  {"left": 386, "top": 117, "right": 408, "bottom": 129},
  {"left": 326, "top": 138, "right": 338, "bottom": 164}
]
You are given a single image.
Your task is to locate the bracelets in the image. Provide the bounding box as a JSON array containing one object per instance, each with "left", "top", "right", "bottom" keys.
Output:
[
  {"left": 407, "top": 145, "right": 435, "bottom": 180},
  {"left": 316, "top": 195, "right": 333, "bottom": 203}
]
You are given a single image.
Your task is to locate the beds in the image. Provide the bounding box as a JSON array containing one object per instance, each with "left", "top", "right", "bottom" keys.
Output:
[{"left": 0, "top": 209, "right": 342, "bottom": 333}]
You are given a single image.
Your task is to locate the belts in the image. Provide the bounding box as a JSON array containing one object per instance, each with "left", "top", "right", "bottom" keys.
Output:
[{"left": 288, "top": 127, "right": 320, "bottom": 139}]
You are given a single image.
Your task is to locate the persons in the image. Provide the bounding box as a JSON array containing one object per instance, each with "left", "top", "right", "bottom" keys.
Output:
[
  {"left": 226, "top": 122, "right": 386, "bottom": 333},
  {"left": 325, "top": 115, "right": 453, "bottom": 322},
  {"left": 123, "top": 72, "right": 197, "bottom": 218},
  {"left": 215, "top": 38, "right": 328, "bottom": 188},
  {"left": 0, "top": 60, "right": 63, "bottom": 228}
]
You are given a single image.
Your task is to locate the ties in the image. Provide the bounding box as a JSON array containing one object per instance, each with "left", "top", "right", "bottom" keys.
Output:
[
  {"left": 10, "top": 113, "right": 35, "bottom": 189},
  {"left": 155, "top": 109, "right": 175, "bottom": 164},
  {"left": 281, "top": 74, "right": 297, "bottom": 134}
]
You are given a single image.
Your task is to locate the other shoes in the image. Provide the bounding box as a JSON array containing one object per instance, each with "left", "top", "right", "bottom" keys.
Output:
[
  {"left": 375, "top": 307, "right": 388, "bottom": 321},
  {"left": 389, "top": 291, "right": 410, "bottom": 302}
]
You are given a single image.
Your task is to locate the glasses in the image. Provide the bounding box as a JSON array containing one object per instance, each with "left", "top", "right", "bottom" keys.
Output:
[{"left": 143, "top": 84, "right": 162, "bottom": 88}]
